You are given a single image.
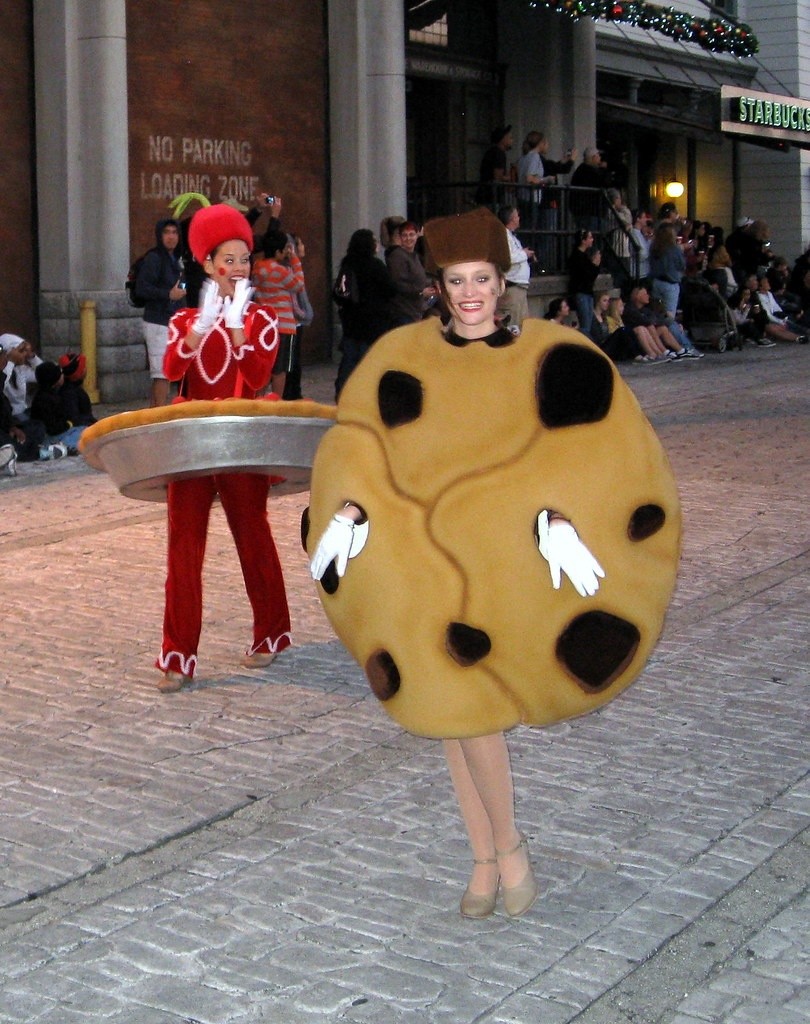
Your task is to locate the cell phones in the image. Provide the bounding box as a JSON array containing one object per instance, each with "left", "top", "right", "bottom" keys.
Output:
[{"left": 265, "top": 196, "right": 275, "bottom": 204}]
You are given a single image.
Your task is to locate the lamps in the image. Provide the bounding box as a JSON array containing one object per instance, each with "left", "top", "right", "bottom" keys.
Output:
[{"left": 666, "top": 174, "right": 685, "bottom": 197}]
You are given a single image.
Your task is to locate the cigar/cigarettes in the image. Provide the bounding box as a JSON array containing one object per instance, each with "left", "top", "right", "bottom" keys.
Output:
[{"left": 532, "top": 253, "right": 537, "bottom": 261}]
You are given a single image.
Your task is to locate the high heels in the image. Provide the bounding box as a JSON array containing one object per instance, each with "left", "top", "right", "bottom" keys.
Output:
[{"left": 460, "top": 832, "right": 538, "bottom": 920}]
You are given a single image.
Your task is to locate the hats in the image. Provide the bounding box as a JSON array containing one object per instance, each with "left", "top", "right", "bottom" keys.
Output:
[
  {"left": 35, "top": 362, "right": 60, "bottom": 386},
  {"left": 424, "top": 208, "right": 514, "bottom": 273},
  {"left": 0, "top": 333, "right": 24, "bottom": 353},
  {"left": 169, "top": 192, "right": 255, "bottom": 266},
  {"left": 59, "top": 352, "right": 86, "bottom": 381},
  {"left": 736, "top": 217, "right": 754, "bottom": 227}
]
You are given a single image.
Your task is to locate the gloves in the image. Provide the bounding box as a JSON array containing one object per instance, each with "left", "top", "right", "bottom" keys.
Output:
[
  {"left": 541, "top": 523, "right": 605, "bottom": 597},
  {"left": 223, "top": 278, "right": 252, "bottom": 328},
  {"left": 310, "top": 513, "right": 355, "bottom": 580},
  {"left": 192, "top": 280, "right": 223, "bottom": 335}
]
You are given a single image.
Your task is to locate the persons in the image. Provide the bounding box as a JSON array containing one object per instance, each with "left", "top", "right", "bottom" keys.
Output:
[
  {"left": 334, "top": 207, "right": 534, "bottom": 407},
  {"left": 133, "top": 193, "right": 313, "bottom": 409},
  {"left": 477, "top": 123, "right": 612, "bottom": 273},
  {"left": 155, "top": 204, "right": 293, "bottom": 693},
  {"left": 0, "top": 333, "right": 98, "bottom": 477},
  {"left": 543, "top": 189, "right": 810, "bottom": 365},
  {"left": 309, "top": 209, "right": 607, "bottom": 920}
]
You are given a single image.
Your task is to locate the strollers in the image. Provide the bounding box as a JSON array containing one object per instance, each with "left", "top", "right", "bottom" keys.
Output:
[{"left": 678, "top": 278, "right": 743, "bottom": 354}]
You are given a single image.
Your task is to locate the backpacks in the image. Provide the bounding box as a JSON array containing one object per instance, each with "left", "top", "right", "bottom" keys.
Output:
[
  {"left": 125, "top": 249, "right": 165, "bottom": 308},
  {"left": 332, "top": 259, "right": 370, "bottom": 308}
]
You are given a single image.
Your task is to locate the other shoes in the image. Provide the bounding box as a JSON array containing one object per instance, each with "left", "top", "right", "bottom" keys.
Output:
[
  {"left": 633, "top": 346, "right": 705, "bottom": 365},
  {"left": 159, "top": 671, "right": 193, "bottom": 693},
  {"left": 0, "top": 444, "right": 19, "bottom": 477},
  {"left": 244, "top": 653, "right": 278, "bottom": 668},
  {"left": 758, "top": 338, "right": 776, "bottom": 348},
  {"left": 47, "top": 444, "right": 67, "bottom": 459},
  {"left": 796, "top": 335, "right": 808, "bottom": 344}
]
